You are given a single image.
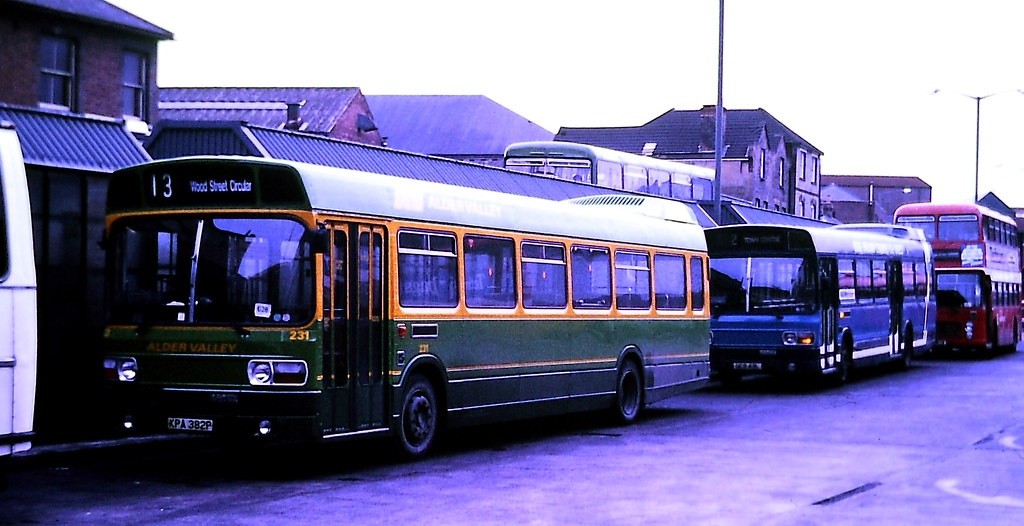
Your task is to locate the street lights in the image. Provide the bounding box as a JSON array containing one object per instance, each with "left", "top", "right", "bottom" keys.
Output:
[{"left": 933, "top": 87, "right": 1024, "bottom": 203}]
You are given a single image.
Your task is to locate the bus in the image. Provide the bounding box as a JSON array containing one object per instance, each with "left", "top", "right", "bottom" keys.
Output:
[
  {"left": 503, "top": 141, "right": 716, "bottom": 201},
  {"left": 0, "top": 121, "right": 38, "bottom": 457},
  {"left": 892, "top": 202, "right": 1024, "bottom": 358},
  {"left": 705, "top": 223, "right": 937, "bottom": 386},
  {"left": 101, "top": 155, "right": 711, "bottom": 460}
]
